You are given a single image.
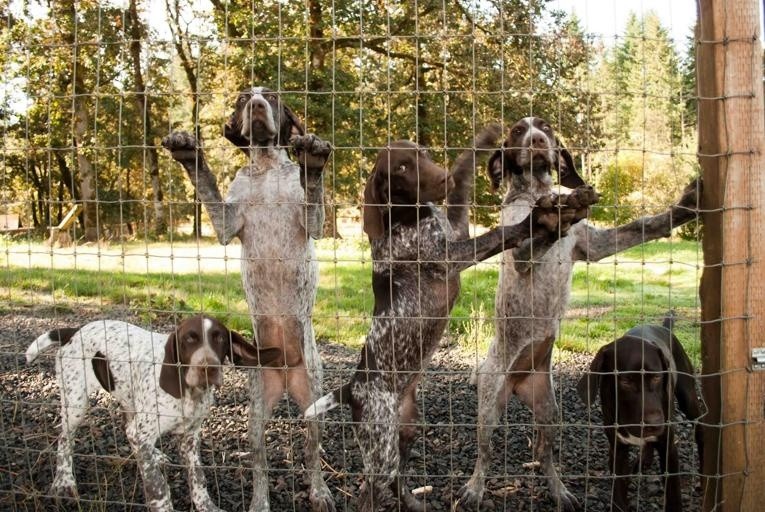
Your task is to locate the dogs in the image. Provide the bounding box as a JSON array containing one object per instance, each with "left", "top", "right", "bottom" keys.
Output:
[
  {"left": 161, "top": 86, "right": 336, "bottom": 512},
  {"left": 304, "top": 121, "right": 575, "bottom": 511},
  {"left": 456, "top": 117, "right": 702, "bottom": 512},
  {"left": 24, "top": 316, "right": 280, "bottom": 512},
  {"left": 578, "top": 310, "right": 705, "bottom": 512}
]
